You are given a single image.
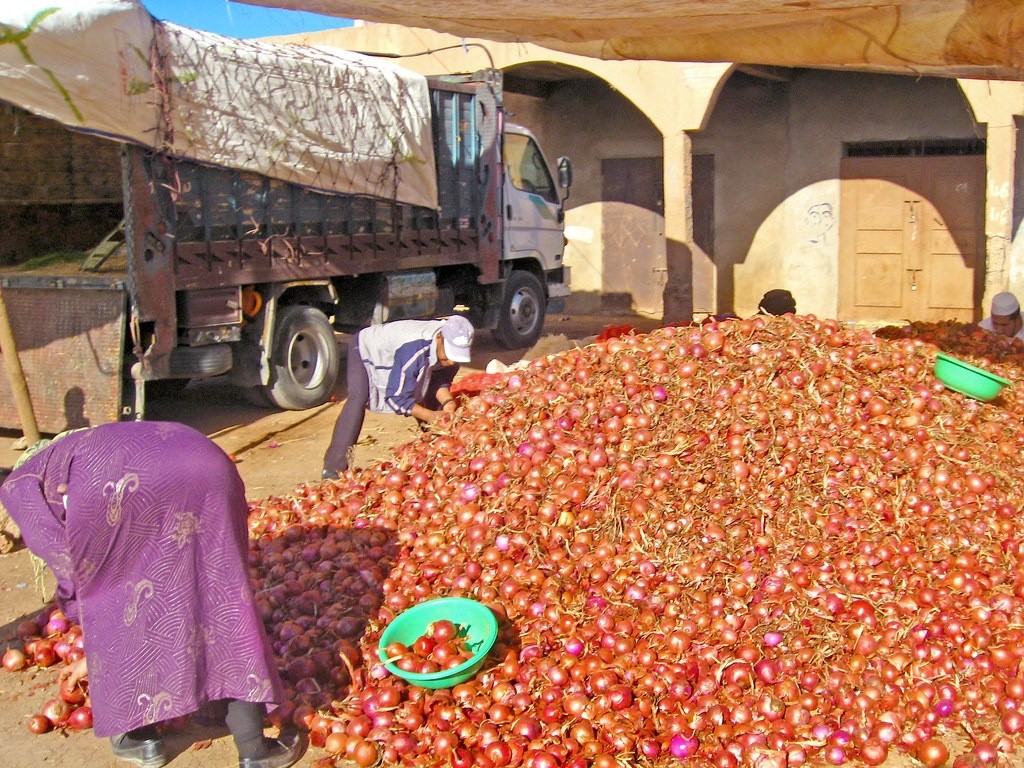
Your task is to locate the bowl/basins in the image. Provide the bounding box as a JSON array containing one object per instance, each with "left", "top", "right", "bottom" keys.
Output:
[
  {"left": 378, "top": 597, "right": 498, "bottom": 689},
  {"left": 934, "top": 353, "right": 1011, "bottom": 402}
]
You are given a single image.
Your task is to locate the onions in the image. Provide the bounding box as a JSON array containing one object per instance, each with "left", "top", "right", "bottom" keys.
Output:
[{"left": 2, "top": 310, "right": 1024, "bottom": 768}]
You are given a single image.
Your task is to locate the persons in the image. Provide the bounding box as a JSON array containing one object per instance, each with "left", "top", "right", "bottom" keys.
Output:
[
  {"left": 0, "top": 421, "right": 302, "bottom": 768},
  {"left": 322, "top": 314, "right": 474, "bottom": 480},
  {"left": 978, "top": 292, "right": 1024, "bottom": 340}
]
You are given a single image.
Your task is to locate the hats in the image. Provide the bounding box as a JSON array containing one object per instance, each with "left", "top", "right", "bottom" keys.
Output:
[
  {"left": 992, "top": 292, "right": 1018, "bottom": 316},
  {"left": 441, "top": 315, "right": 475, "bottom": 363}
]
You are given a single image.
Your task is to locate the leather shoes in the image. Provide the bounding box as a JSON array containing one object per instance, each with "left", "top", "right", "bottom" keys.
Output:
[
  {"left": 111, "top": 725, "right": 167, "bottom": 768},
  {"left": 239, "top": 724, "right": 301, "bottom": 768}
]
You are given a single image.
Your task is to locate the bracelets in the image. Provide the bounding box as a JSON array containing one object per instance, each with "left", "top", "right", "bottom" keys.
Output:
[{"left": 442, "top": 399, "right": 455, "bottom": 410}]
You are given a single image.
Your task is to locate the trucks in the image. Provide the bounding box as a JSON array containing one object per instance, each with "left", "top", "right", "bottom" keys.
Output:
[{"left": 0, "top": 0, "right": 574, "bottom": 442}]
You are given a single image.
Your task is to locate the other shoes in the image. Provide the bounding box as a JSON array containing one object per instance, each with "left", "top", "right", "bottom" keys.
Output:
[{"left": 322, "top": 468, "right": 340, "bottom": 480}]
